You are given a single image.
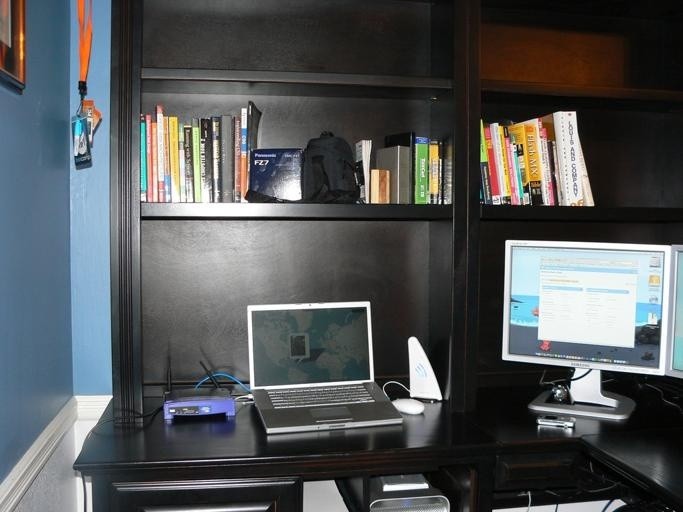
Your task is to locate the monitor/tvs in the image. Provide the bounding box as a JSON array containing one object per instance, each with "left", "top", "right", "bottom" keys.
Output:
[
  {"left": 501, "top": 239, "right": 670, "bottom": 422},
  {"left": 669, "top": 242, "right": 683, "bottom": 378}
]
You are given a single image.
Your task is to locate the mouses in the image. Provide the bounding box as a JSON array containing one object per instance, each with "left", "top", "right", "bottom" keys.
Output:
[{"left": 394, "top": 398, "right": 425, "bottom": 415}]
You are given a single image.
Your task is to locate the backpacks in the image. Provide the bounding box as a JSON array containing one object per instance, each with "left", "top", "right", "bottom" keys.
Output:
[{"left": 300, "top": 131, "right": 360, "bottom": 203}]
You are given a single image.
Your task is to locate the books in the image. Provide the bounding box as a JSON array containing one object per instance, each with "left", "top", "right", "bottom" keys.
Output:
[
  {"left": 140, "top": 99, "right": 262, "bottom": 204},
  {"left": 357, "top": 137, "right": 452, "bottom": 204},
  {"left": 480, "top": 110, "right": 594, "bottom": 208}
]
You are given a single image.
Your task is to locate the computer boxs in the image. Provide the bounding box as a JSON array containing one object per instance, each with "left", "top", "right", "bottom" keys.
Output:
[{"left": 357, "top": 471, "right": 451, "bottom": 512}]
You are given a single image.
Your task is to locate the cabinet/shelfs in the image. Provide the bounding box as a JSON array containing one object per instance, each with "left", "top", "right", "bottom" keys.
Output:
[{"left": 103, "top": 0, "right": 683, "bottom": 429}]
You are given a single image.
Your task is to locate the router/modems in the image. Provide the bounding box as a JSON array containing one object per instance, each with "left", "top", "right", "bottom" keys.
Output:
[{"left": 161, "top": 360, "right": 237, "bottom": 426}]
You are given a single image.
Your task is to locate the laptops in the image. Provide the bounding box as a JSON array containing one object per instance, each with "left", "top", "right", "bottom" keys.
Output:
[{"left": 246, "top": 301, "right": 404, "bottom": 436}]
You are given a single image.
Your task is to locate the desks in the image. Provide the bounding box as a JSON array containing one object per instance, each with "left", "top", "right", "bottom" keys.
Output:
[{"left": 72, "top": 387, "right": 683, "bottom": 510}]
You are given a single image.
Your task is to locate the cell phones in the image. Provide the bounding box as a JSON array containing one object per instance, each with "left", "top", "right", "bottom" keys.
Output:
[{"left": 535, "top": 413, "right": 577, "bottom": 428}]
